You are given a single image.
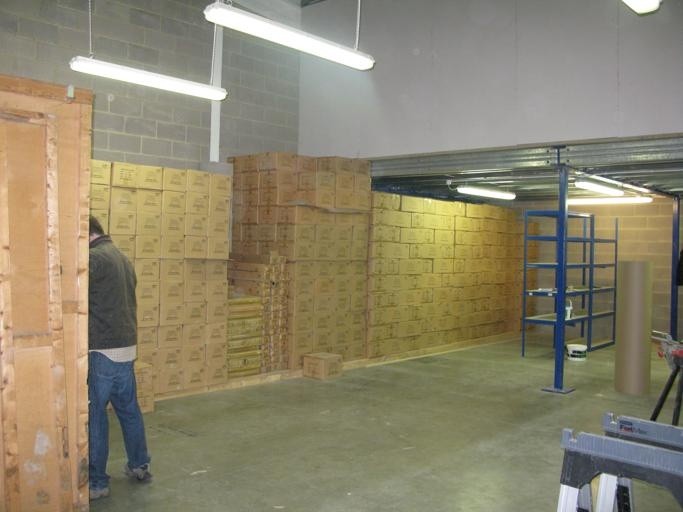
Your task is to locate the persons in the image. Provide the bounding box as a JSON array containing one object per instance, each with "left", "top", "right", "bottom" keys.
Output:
[{"left": 89, "top": 216, "right": 152, "bottom": 500}]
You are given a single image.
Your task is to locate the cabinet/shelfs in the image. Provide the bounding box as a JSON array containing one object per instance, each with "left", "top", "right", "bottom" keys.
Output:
[{"left": 520, "top": 209, "right": 619, "bottom": 357}]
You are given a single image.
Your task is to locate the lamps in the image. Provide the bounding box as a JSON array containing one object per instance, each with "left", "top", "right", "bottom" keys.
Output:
[
  {"left": 202, "top": 2, "right": 375, "bottom": 72},
  {"left": 69, "top": 2, "right": 227, "bottom": 100}
]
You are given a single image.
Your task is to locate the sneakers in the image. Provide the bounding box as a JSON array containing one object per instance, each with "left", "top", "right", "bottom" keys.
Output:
[
  {"left": 89, "top": 481, "right": 111, "bottom": 500},
  {"left": 124, "top": 462, "right": 154, "bottom": 483}
]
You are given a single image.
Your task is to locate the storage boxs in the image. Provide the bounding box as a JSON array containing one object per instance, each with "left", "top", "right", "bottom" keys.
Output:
[
  {"left": 228, "top": 155, "right": 373, "bottom": 380},
  {"left": 367, "top": 189, "right": 539, "bottom": 358},
  {"left": 89, "top": 157, "right": 234, "bottom": 416}
]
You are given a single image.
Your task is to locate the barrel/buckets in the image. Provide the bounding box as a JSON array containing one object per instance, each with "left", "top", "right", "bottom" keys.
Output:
[{"left": 568, "top": 343, "right": 589, "bottom": 360}]
